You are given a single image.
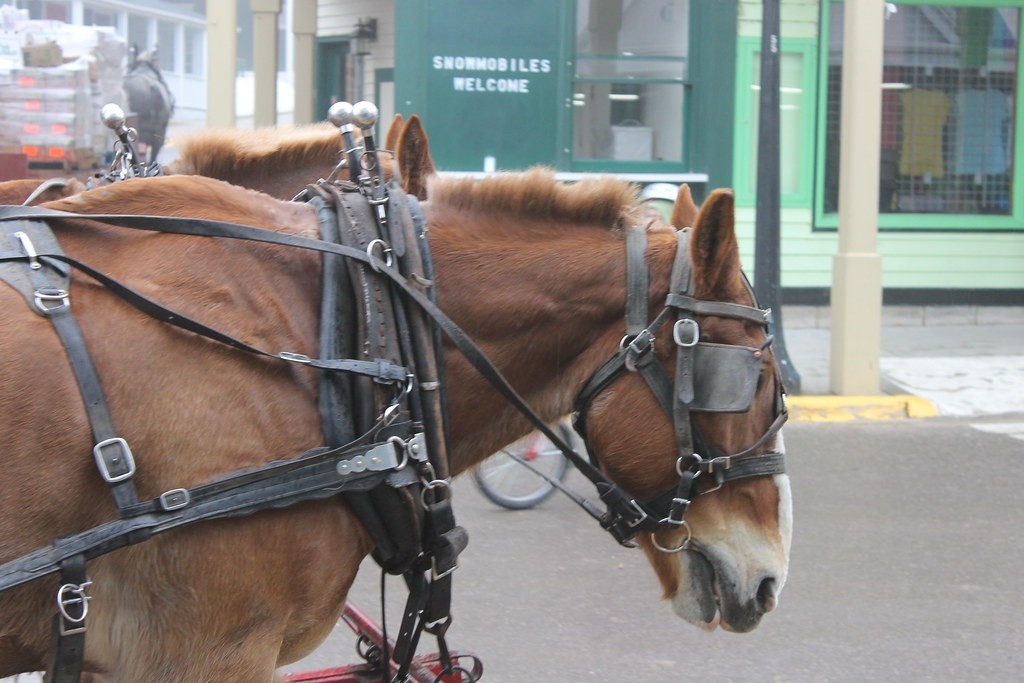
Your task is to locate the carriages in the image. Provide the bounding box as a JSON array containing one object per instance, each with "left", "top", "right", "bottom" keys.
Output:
[{"left": 0, "top": 3, "right": 175, "bottom": 170}]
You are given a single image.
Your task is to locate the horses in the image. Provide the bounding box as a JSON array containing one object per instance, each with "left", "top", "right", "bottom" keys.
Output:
[
  {"left": 123, "top": 44, "right": 174, "bottom": 166},
  {"left": 0, "top": 114, "right": 793, "bottom": 683}
]
